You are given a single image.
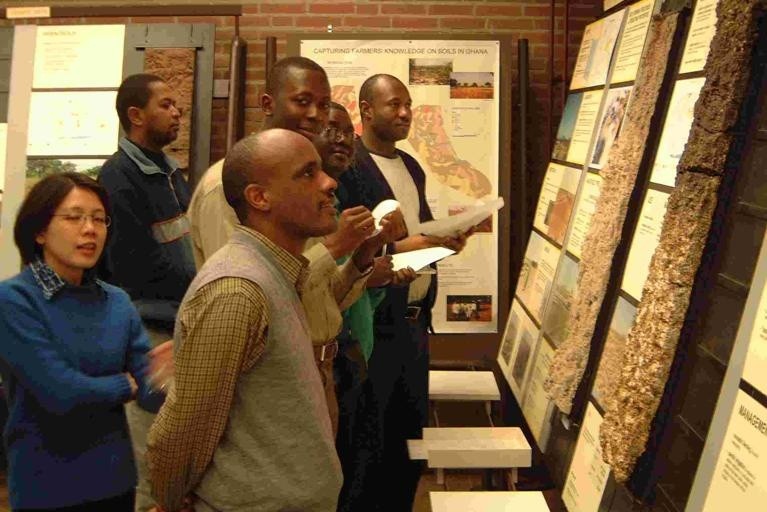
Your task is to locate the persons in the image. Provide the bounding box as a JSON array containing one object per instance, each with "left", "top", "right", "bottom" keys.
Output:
[
  {"left": 97, "top": 72, "right": 197, "bottom": 511},
  {"left": 0, "top": 172, "right": 173, "bottom": 512},
  {"left": 144, "top": 127, "right": 345, "bottom": 510},
  {"left": 604, "top": 91, "right": 630, "bottom": 131},
  {"left": 450, "top": 299, "right": 483, "bottom": 319},
  {"left": 312, "top": 101, "right": 423, "bottom": 511},
  {"left": 185, "top": 54, "right": 409, "bottom": 447},
  {"left": 333, "top": 72, "right": 480, "bottom": 512}
]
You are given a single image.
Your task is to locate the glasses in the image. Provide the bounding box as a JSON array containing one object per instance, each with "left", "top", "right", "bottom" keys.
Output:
[
  {"left": 52, "top": 212, "right": 112, "bottom": 228},
  {"left": 322, "top": 125, "right": 354, "bottom": 142}
]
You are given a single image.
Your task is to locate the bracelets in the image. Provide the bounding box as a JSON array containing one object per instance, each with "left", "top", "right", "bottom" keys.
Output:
[{"left": 352, "top": 251, "right": 374, "bottom": 276}]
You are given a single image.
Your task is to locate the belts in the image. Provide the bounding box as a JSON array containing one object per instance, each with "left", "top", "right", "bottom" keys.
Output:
[
  {"left": 313, "top": 337, "right": 338, "bottom": 362},
  {"left": 384, "top": 302, "right": 426, "bottom": 319}
]
